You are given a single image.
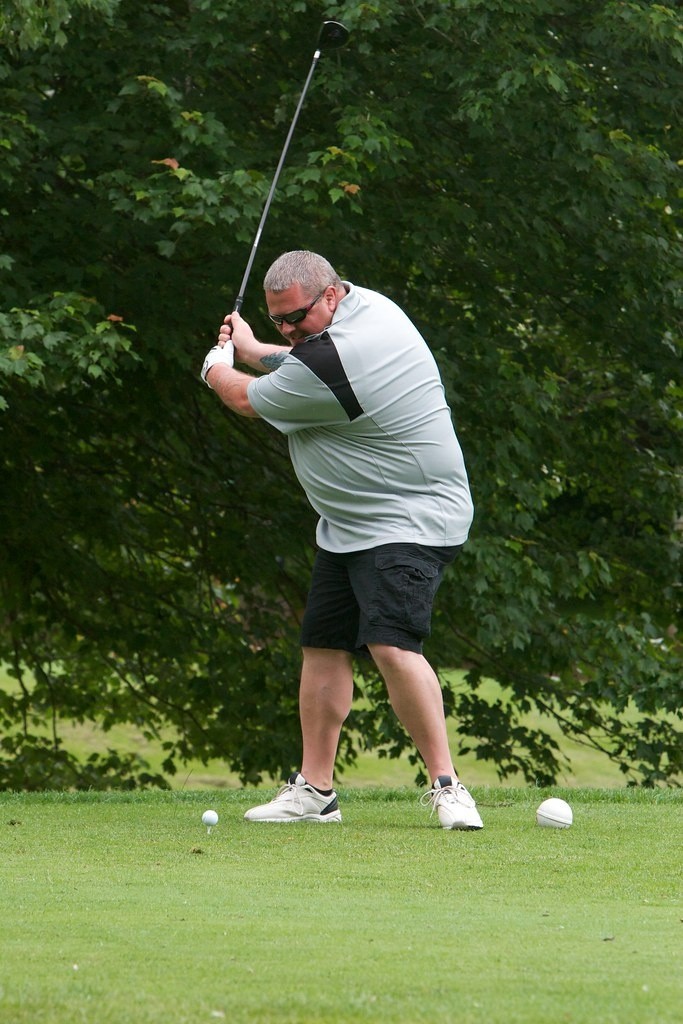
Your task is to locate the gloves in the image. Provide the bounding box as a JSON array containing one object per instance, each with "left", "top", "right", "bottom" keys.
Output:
[{"left": 200, "top": 340, "right": 235, "bottom": 390}]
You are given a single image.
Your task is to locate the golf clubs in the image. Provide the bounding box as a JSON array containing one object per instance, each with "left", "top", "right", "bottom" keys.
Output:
[{"left": 228, "top": 21, "right": 350, "bottom": 332}]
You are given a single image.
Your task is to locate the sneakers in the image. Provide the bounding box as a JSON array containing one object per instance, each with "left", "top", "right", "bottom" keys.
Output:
[
  {"left": 244, "top": 772, "right": 341, "bottom": 823},
  {"left": 420, "top": 776, "right": 484, "bottom": 829}
]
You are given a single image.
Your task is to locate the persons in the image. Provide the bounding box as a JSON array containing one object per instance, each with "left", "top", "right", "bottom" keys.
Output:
[{"left": 200, "top": 251, "right": 480, "bottom": 831}]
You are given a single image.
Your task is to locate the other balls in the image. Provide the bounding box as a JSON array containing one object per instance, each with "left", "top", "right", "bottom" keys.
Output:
[{"left": 536, "top": 797, "right": 573, "bottom": 829}]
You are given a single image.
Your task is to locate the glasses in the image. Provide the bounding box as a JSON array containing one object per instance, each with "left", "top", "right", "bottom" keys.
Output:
[{"left": 268, "top": 291, "right": 324, "bottom": 325}]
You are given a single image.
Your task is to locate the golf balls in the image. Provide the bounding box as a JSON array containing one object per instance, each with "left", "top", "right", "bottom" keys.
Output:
[{"left": 202, "top": 810, "right": 219, "bottom": 826}]
